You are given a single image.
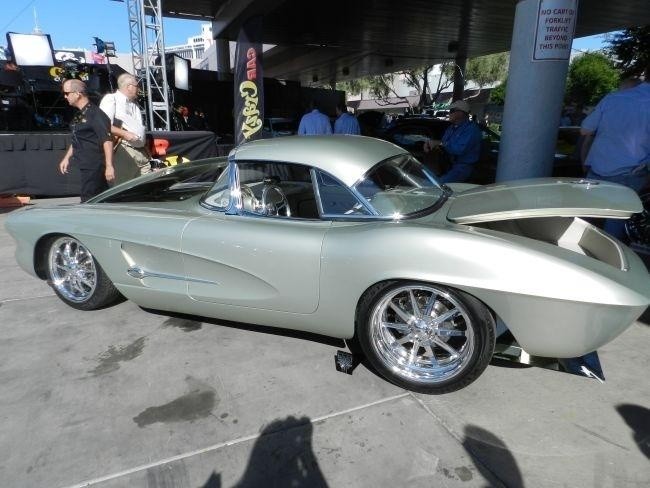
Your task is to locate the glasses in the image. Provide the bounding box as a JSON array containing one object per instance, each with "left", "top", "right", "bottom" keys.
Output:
[{"left": 62, "top": 90, "right": 84, "bottom": 97}]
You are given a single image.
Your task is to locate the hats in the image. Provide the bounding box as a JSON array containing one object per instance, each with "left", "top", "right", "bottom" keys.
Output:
[{"left": 448, "top": 100, "right": 470, "bottom": 109}]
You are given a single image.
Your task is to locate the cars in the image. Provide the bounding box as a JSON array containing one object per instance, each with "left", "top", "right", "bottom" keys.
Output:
[
  {"left": 0, "top": 122, "right": 649, "bottom": 398},
  {"left": 553, "top": 124, "right": 584, "bottom": 152},
  {"left": 623, "top": 182, "right": 650, "bottom": 271},
  {"left": 421, "top": 109, "right": 473, "bottom": 122},
  {"left": 263, "top": 116, "right": 294, "bottom": 138}
]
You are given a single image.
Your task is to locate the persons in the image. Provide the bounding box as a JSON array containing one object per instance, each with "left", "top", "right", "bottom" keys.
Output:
[
  {"left": 578, "top": 64, "right": 649, "bottom": 241},
  {"left": 298, "top": 100, "right": 482, "bottom": 185},
  {"left": 174, "top": 100, "right": 210, "bottom": 132},
  {"left": 581, "top": 77, "right": 642, "bottom": 178},
  {"left": 58, "top": 79, "right": 116, "bottom": 202},
  {"left": 98, "top": 73, "right": 152, "bottom": 189}
]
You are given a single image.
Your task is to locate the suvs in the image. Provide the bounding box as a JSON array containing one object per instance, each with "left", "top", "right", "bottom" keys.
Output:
[{"left": 358, "top": 109, "right": 580, "bottom": 184}]
